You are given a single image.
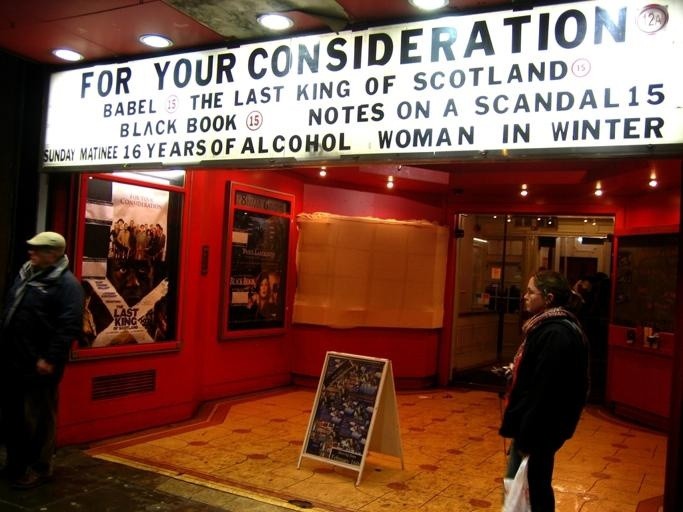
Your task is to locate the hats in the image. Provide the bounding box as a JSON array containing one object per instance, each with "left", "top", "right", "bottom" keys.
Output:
[{"left": 27, "top": 232, "right": 65, "bottom": 247}]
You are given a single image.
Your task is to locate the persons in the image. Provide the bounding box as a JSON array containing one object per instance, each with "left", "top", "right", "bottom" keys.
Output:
[
  {"left": 91, "top": 261, "right": 157, "bottom": 343},
  {"left": 486, "top": 267, "right": 591, "bottom": 511},
  {"left": 108, "top": 218, "right": 165, "bottom": 261},
  {"left": 246, "top": 271, "right": 275, "bottom": 320},
  {"left": 0, "top": 227, "right": 85, "bottom": 487}
]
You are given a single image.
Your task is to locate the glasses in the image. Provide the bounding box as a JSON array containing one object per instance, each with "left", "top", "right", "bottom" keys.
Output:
[{"left": 526, "top": 289, "right": 550, "bottom": 298}]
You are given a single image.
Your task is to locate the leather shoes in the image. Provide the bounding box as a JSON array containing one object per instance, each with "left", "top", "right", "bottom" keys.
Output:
[{"left": 12, "top": 469, "right": 52, "bottom": 488}]
